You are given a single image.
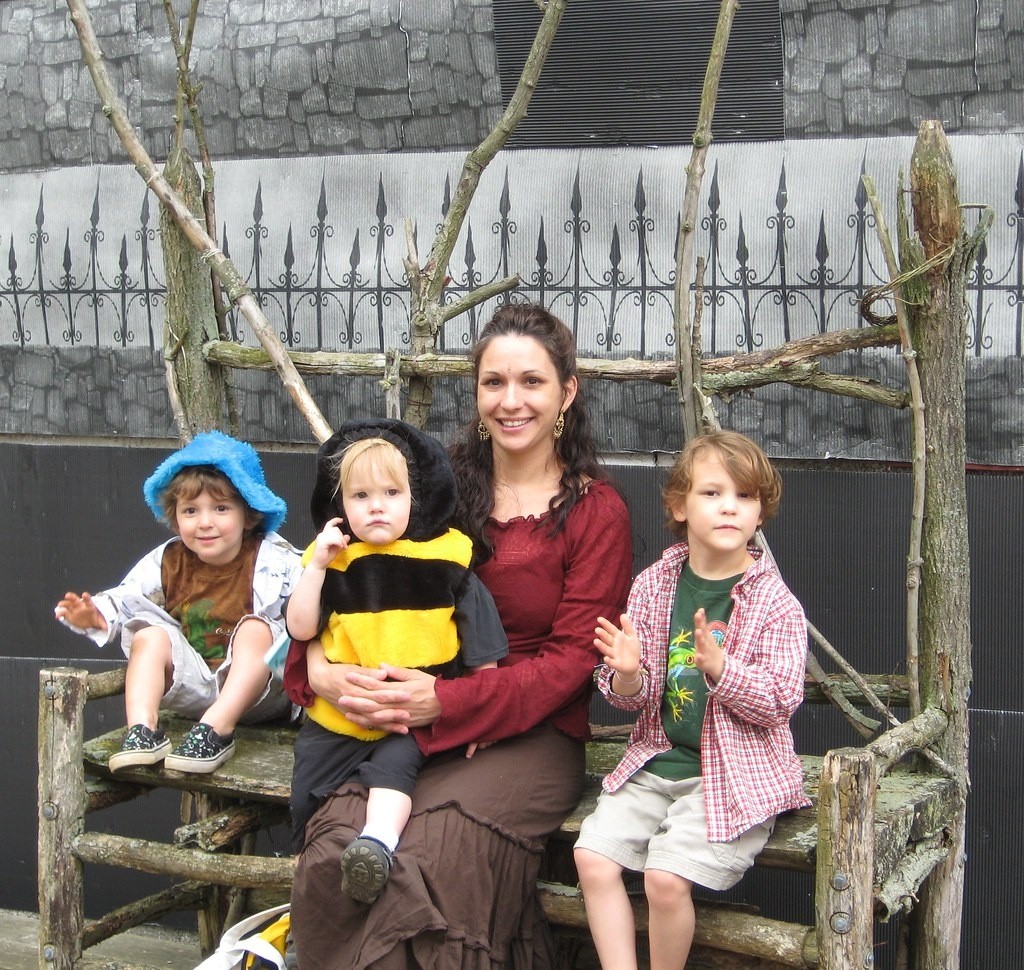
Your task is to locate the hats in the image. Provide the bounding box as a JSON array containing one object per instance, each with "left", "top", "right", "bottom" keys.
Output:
[{"left": 143, "top": 430, "right": 287, "bottom": 541}]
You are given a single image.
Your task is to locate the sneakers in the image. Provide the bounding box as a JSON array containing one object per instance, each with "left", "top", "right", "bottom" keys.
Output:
[
  {"left": 109, "top": 724, "right": 174, "bottom": 772},
  {"left": 164, "top": 722, "right": 236, "bottom": 774}
]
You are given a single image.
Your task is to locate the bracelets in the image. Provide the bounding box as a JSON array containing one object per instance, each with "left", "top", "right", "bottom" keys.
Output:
[{"left": 614, "top": 670, "right": 640, "bottom": 684}]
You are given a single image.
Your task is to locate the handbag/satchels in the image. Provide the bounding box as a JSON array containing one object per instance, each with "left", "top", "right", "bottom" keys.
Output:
[{"left": 191, "top": 903, "right": 299, "bottom": 970}]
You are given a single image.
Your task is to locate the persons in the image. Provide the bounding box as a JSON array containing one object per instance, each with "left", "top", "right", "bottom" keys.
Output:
[
  {"left": 572, "top": 428, "right": 814, "bottom": 970},
  {"left": 281, "top": 304, "right": 631, "bottom": 970},
  {"left": 54, "top": 431, "right": 303, "bottom": 774}
]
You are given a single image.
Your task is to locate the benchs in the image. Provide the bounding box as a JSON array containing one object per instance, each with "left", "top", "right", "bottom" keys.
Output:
[{"left": 39, "top": 113, "right": 995, "bottom": 970}]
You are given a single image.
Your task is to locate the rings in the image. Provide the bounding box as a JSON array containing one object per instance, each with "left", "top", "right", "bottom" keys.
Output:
[{"left": 368, "top": 726, "right": 376, "bottom": 730}]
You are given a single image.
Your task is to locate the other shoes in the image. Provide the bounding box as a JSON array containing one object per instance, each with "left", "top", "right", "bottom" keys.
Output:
[
  {"left": 284, "top": 927, "right": 298, "bottom": 968},
  {"left": 341, "top": 836, "right": 395, "bottom": 904}
]
